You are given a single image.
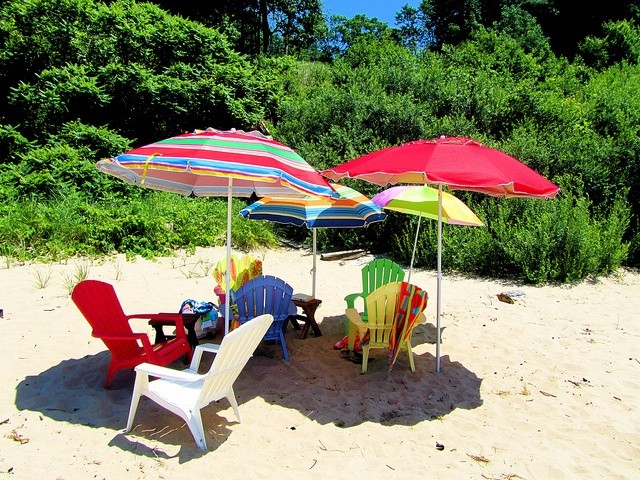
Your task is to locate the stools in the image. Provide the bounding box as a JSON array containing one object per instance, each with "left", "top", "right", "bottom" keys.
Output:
[
  {"left": 288, "top": 298, "right": 322, "bottom": 339},
  {"left": 149, "top": 312, "right": 200, "bottom": 348}
]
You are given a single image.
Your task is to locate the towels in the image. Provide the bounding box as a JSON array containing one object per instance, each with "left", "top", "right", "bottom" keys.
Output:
[{"left": 291, "top": 292, "right": 314, "bottom": 303}]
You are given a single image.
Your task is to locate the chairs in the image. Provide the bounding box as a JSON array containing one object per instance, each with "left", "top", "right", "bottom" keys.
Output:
[
  {"left": 345, "top": 282, "right": 428, "bottom": 373},
  {"left": 120, "top": 314, "right": 276, "bottom": 451},
  {"left": 345, "top": 259, "right": 405, "bottom": 335},
  {"left": 70, "top": 281, "right": 192, "bottom": 389},
  {"left": 235, "top": 278, "right": 294, "bottom": 359}
]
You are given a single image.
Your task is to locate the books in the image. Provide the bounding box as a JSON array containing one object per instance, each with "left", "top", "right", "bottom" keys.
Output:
[{"left": 293, "top": 293, "right": 313, "bottom": 303}]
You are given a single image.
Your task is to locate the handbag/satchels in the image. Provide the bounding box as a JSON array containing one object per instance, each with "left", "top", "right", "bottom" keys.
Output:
[{"left": 173, "top": 299, "right": 219, "bottom": 340}]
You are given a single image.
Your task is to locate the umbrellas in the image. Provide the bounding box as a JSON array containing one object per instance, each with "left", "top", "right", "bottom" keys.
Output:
[
  {"left": 98, "top": 122, "right": 341, "bottom": 337},
  {"left": 239, "top": 184, "right": 388, "bottom": 335},
  {"left": 372, "top": 179, "right": 485, "bottom": 284},
  {"left": 212, "top": 253, "right": 262, "bottom": 332},
  {"left": 388, "top": 215, "right": 434, "bottom": 374},
  {"left": 321, "top": 135, "right": 560, "bottom": 372}
]
